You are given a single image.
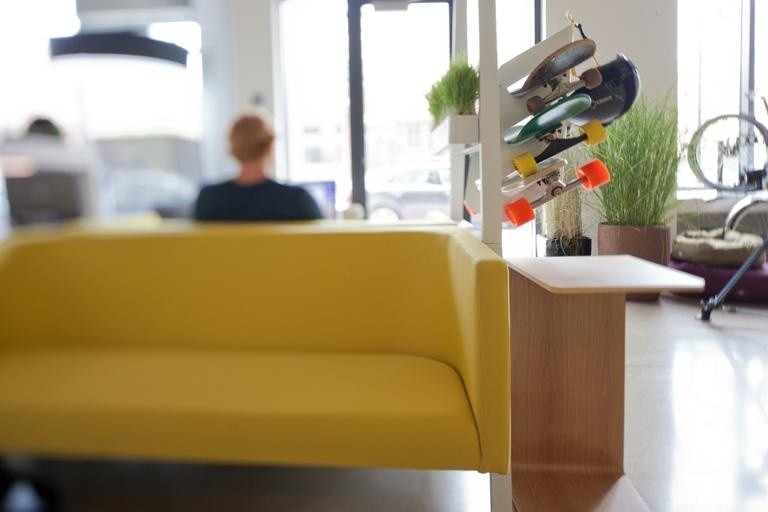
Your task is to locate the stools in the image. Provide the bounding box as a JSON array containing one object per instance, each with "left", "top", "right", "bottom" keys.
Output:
[{"left": 669, "top": 193, "right": 768, "bottom": 300}]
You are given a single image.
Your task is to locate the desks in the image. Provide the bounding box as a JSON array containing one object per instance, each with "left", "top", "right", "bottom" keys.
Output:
[{"left": 505, "top": 253, "right": 707, "bottom": 512}]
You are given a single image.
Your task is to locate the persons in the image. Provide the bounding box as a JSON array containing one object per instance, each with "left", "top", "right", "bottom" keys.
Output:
[{"left": 188, "top": 112, "right": 323, "bottom": 221}]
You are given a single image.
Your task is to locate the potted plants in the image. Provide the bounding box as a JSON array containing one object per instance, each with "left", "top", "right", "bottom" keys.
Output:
[
  {"left": 424, "top": 56, "right": 478, "bottom": 155},
  {"left": 542, "top": 158, "right": 591, "bottom": 256},
  {"left": 587, "top": 93, "right": 679, "bottom": 302}
]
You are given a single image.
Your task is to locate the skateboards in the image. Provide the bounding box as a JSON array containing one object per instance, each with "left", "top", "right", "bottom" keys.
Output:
[{"left": 464, "top": 39, "right": 609, "bottom": 231}]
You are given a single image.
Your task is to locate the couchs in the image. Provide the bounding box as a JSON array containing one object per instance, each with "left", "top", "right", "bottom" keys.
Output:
[{"left": 1, "top": 215, "right": 511, "bottom": 475}]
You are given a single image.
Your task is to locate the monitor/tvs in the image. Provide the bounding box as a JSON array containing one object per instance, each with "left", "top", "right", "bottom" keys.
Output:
[{"left": 293, "top": 180, "right": 336, "bottom": 220}]
